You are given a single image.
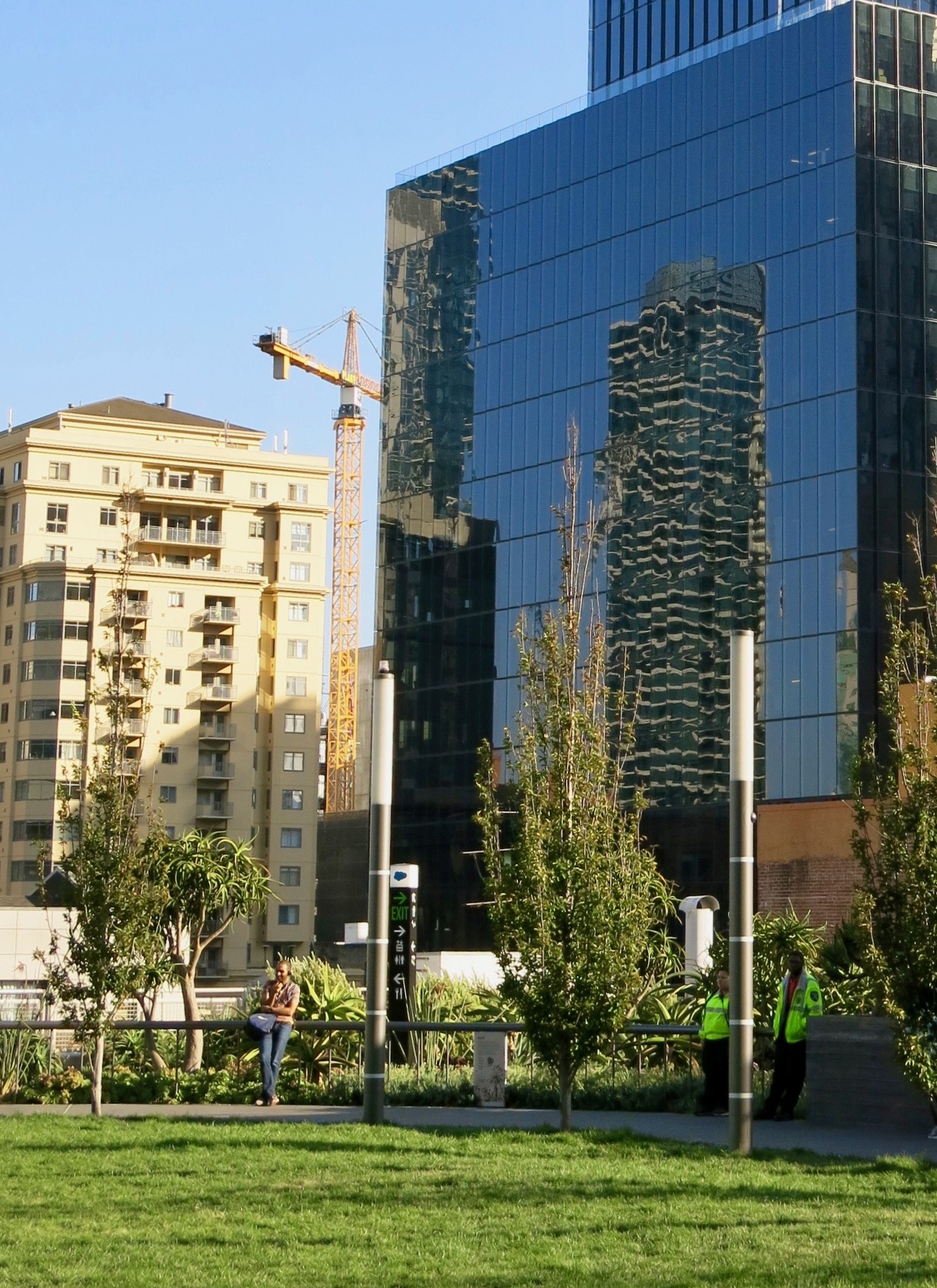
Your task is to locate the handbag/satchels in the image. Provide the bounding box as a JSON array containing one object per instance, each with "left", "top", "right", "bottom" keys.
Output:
[{"left": 246, "top": 1007, "right": 277, "bottom": 1036}]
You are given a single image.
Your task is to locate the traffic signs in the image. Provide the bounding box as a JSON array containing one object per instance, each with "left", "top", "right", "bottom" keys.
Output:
[{"left": 385, "top": 862, "right": 419, "bottom": 1023}]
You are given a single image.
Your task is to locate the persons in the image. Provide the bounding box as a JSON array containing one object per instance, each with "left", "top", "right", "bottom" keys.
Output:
[
  {"left": 694, "top": 967, "right": 729, "bottom": 1116},
  {"left": 753, "top": 951, "right": 823, "bottom": 1122},
  {"left": 255, "top": 960, "right": 300, "bottom": 1106}
]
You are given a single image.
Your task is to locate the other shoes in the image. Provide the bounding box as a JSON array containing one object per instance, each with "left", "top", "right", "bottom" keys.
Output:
[
  {"left": 712, "top": 1107, "right": 730, "bottom": 1116},
  {"left": 270, "top": 1096, "right": 279, "bottom": 1107},
  {"left": 694, "top": 1107, "right": 712, "bottom": 1115},
  {"left": 753, "top": 1108, "right": 774, "bottom": 1120},
  {"left": 255, "top": 1097, "right": 270, "bottom": 1106},
  {"left": 774, "top": 1109, "right": 794, "bottom": 1122}
]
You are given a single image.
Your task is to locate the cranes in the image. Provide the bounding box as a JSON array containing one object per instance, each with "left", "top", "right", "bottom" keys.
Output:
[{"left": 252, "top": 306, "right": 384, "bottom": 823}]
